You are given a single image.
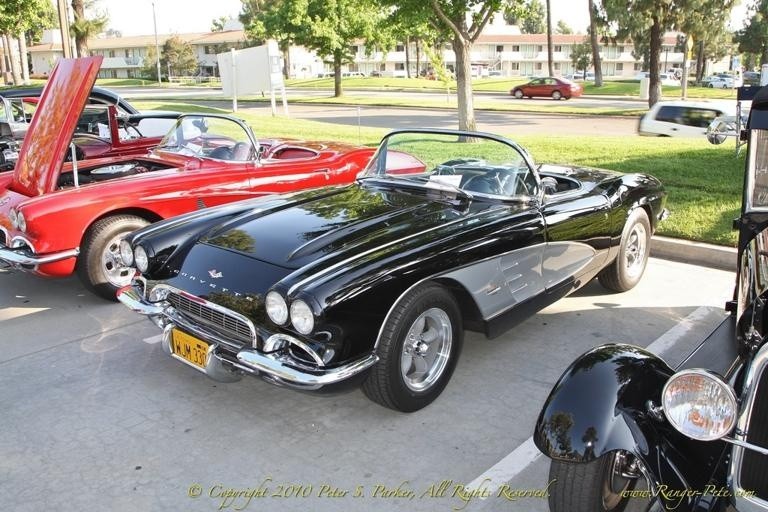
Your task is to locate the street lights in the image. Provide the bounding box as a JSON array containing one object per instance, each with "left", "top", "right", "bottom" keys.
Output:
[{"left": 150, "top": 1, "right": 163, "bottom": 83}]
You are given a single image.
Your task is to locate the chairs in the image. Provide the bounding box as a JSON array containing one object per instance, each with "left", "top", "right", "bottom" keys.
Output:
[
  {"left": 435, "top": 158, "right": 509, "bottom": 194},
  {"left": 510, "top": 170, "right": 580, "bottom": 199},
  {"left": 262, "top": 142, "right": 317, "bottom": 161},
  {"left": 239, "top": 138, "right": 270, "bottom": 157}
]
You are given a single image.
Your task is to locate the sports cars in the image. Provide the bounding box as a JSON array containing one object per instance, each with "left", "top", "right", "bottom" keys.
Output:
[
  {"left": 0, "top": 55, "right": 428, "bottom": 305},
  {"left": 116, "top": 127, "right": 669, "bottom": 412}
]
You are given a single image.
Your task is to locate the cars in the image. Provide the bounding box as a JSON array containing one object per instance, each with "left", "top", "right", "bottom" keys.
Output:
[
  {"left": 508, "top": 74, "right": 582, "bottom": 100},
  {"left": 316, "top": 71, "right": 335, "bottom": 78},
  {"left": 631, "top": 66, "right": 762, "bottom": 88},
  {"left": 0, "top": 84, "right": 143, "bottom": 130},
  {"left": 0, "top": 94, "right": 207, "bottom": 174},
  {"left": 369, "top": 71, "right": 384, "bottom": 76},
  {"left": 563, "top": 69, "right": 594, "bottom": 80},
  {"left": 342, "top": 72, "right": 366, "bottom": 77},
  {"left": 531, "top": 80, "right": 768, "bottom": 510},
  {"left": 424, "top": 68, "right": 454, "bottom": 81}
]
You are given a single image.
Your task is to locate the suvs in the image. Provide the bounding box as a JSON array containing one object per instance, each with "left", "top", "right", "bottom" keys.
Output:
[{"left": 636, "top": 98, "right": 749, "bottom": 138}]
